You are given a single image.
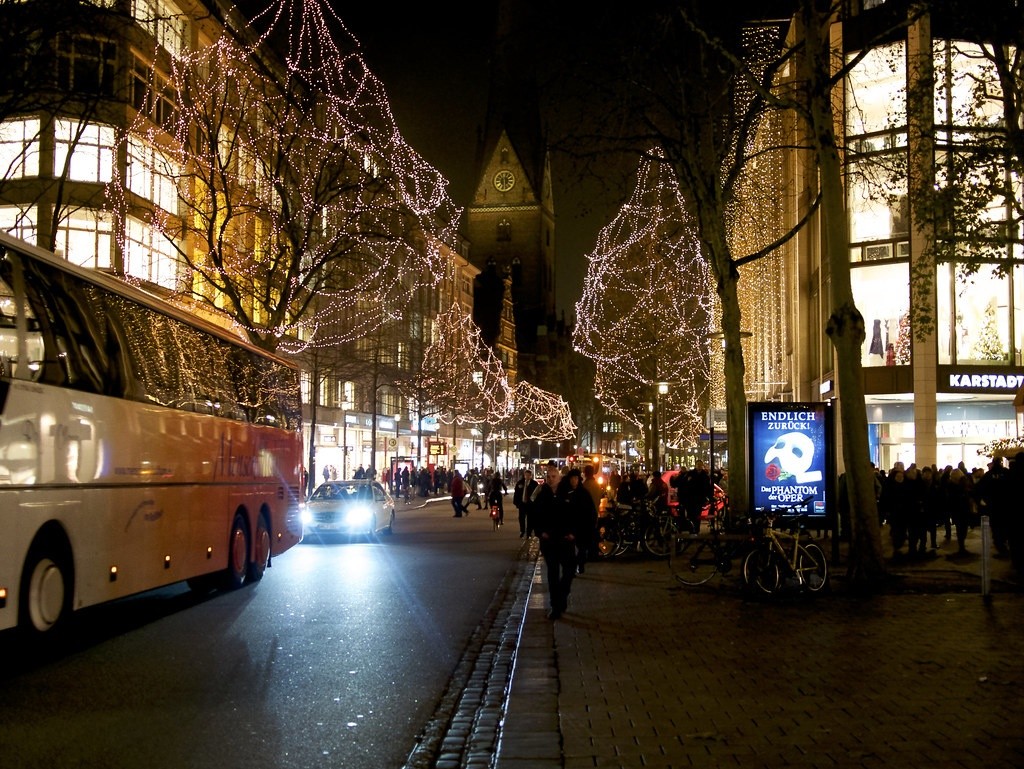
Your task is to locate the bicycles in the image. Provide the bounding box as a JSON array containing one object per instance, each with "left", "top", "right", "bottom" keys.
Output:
[
  {"left": 491, "top": 497, "right": 500, "bottom": 531},
  {"left": 671, "top": 493, "right": 829, "bottom": 598},
  {"left": 596, "top": 496, "right": 686, "bottom": 561}
]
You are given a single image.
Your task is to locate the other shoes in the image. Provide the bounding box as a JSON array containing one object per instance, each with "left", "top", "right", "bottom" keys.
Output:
[
  {"left": 527, "top": 533, "right": 531, "bottom": 540},
  {"left": 931, "top": 544, "right": 939, "bottom": 548},
  {"left": 573, "top": 574, "right": 577, "bottom": 579},
  {"left": 560, "top": 599, "right": 568, "bottom": 613},
  {"left": 520, "top": 531, "right": 525, "bottom": 538},
  {"left": 578, "top": 567, "right": 584, "bottom": 574},
  {"left": 547, "top": 608, "right": 562, "bottom": 620}
]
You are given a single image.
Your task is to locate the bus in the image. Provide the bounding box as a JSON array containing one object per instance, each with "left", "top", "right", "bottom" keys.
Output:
[
  {"left": 566, "top": 454, "right": 627, "bottom": 491},
  {"left": 533, "top": 458, "right": 566, "bottom": 486},
  {"left": 0, "top": 231, "right": 304, "bottom": 654}
]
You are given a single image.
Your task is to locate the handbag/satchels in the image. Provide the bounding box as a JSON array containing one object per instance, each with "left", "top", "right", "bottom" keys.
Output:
[{"left": 471, "top": 493, "right": 480, "bottom": 505}]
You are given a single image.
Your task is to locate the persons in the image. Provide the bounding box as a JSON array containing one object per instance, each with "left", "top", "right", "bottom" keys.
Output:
[
  {"left": 353, "top": 463, "right": 377, "bottom": 480},
  {"left": 382, "top": 463, "right": 526, "bottom": 518},
  {"left": 488, "top": 472, "right": 509, "bottom": 525},
  {"left": 838, "top": 451, "right": 1024, "bottom": 562},
  {"left": 513, "top": 470, "right": 538, "bottom": 541},
  {"left": 566, "top": 469, "right": 598, "bottom": 575},
  {"left": 531, "top": 462, "right": 579, "bottom": 621},
  {"left": 323, "top": 463, "right": 337, "bottom": 482},
  {"left": 303, "top": 467, "right": 309, "bottom": 496},
  {"left": 583, "top": 460, "right": 729, "bottom": 540}
]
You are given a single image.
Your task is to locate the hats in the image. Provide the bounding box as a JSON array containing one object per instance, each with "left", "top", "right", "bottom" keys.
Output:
[{"left": 548, "top": 460, "right": 557, "bottom": 467}]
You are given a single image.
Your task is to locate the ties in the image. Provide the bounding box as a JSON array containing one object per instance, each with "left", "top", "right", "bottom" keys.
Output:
[{"left": 524, "top": 483, "right": 527, "bottom": 501}]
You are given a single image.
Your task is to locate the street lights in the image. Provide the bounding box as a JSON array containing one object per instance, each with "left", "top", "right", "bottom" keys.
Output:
[
  {"left": 688, "top": 449, "right": 719, "bottom": 467},
  {"left": 433, "top": 423, "right": 442, "bottom": 468},
  {"left": 657, "top": 380, "right": 673, "bottom": 474},
  {"left": 391, "top": 412, "right": 402, "bottom": 470},
  {"left": 516, "top": 438, "right": 522, "bottom": 479},
  {"left": 467, "top": 429, "right": 480, "bottom": 469},
  {"left": 553, "top": 443, "right": 562, "bottom": 458},
  {"left": 536, "top": 439, "right": 542, "bottom": 460},
  {"left": 337, "top": 401, "right": 353, "bottom": 481}
]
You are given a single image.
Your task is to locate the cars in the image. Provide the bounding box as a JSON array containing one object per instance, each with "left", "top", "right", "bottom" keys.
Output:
[{"left": 302, "top": 481, "right": 397, "bottom": 542}]
